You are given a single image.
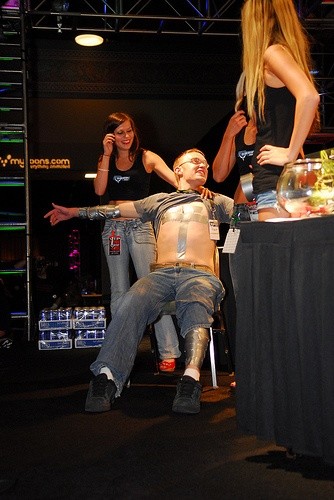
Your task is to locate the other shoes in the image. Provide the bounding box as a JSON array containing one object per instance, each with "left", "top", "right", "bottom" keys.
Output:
[{"left": 160, "top": 359, "right": 176, "bottom": 373}]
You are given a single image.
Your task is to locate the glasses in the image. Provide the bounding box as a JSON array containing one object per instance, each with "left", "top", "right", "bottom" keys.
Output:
[{"left": 177, "top": 160, "right": 208, "bottom": 168}]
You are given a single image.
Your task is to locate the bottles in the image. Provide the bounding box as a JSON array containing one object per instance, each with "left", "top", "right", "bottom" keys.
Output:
[{"left": 277, "top": 158, "right": 334, "bottom": 218}]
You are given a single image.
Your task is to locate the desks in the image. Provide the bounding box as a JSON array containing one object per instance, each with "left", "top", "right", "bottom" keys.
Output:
[{"left": 233, "top": 214, "right": 334, "bottom": 462}]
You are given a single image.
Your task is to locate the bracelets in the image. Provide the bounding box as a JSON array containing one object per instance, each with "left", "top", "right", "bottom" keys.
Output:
[
  {"left": 98, "top": 168, "right": 110, "bottom": 171},
  {"left": 103, "top": 154, "right": 110, "bottom": 158}
]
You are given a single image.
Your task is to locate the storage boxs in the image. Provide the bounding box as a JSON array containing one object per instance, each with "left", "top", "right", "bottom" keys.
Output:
[
  {"left": 39, "top": 319, "right": 72, "bottom": 331},
  {"left": 72, "top": 318, "right": 106, "bottom": 329},
  {"left": 38, "top": 339, "right": 72, "bottom": 350},
  {"left": 75, "top": 338, "right": 104, "bottom": 349}
]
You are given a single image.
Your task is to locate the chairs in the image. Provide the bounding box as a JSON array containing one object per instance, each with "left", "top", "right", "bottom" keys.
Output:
[{"left": 126, "top": 248, "right": 233, "bottom": 389}]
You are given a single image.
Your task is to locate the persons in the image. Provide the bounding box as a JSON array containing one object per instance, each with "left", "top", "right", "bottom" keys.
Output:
[
  {"left": 44, "top": 149, "right": 255, "bottom": 414},
  {"left": 94, "top": 111, "right": 212, "bottom": 371},
  {"left": 213, "top": 0, "right": 320, "bottom": 389}
]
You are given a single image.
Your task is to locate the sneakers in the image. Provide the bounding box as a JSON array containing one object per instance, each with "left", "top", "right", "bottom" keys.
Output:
[
  {"left": 84, "top": 373, "right": 118, "bottom": 411},
  {"left": 173, "top": 375, "right": 203, "bottom": 412}
]
